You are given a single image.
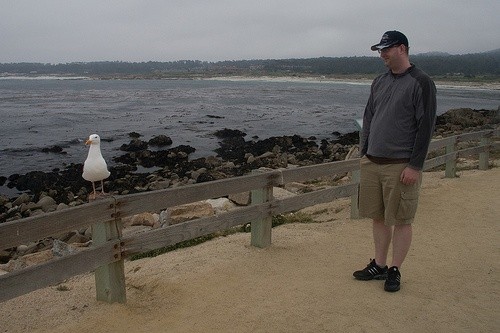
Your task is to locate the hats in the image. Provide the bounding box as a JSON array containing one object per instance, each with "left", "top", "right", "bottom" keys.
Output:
[{"left": 370, "top": 30, "right": 409, "bottom": 51}]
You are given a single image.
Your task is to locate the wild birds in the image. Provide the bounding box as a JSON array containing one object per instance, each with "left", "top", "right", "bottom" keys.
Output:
[{"left": 81, "top": 133, "right": 111, "bottom": 201}]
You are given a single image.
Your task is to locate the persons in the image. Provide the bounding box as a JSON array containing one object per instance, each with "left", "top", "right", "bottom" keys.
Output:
[{"left": 352, "top": 30, "right": 437, "bottom": 293}]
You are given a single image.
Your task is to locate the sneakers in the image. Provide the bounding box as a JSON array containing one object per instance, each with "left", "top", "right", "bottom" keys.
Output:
[
  {"left": 352, "top": 259, "right": 389, "bottom": 280},
  {"left": 384, "top": 266, "right": 401, "bottom": 292}
]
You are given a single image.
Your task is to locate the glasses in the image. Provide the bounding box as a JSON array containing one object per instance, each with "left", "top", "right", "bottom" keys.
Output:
[{"left": 378, "top": 45, "right": 398, "bottom": 54}]
